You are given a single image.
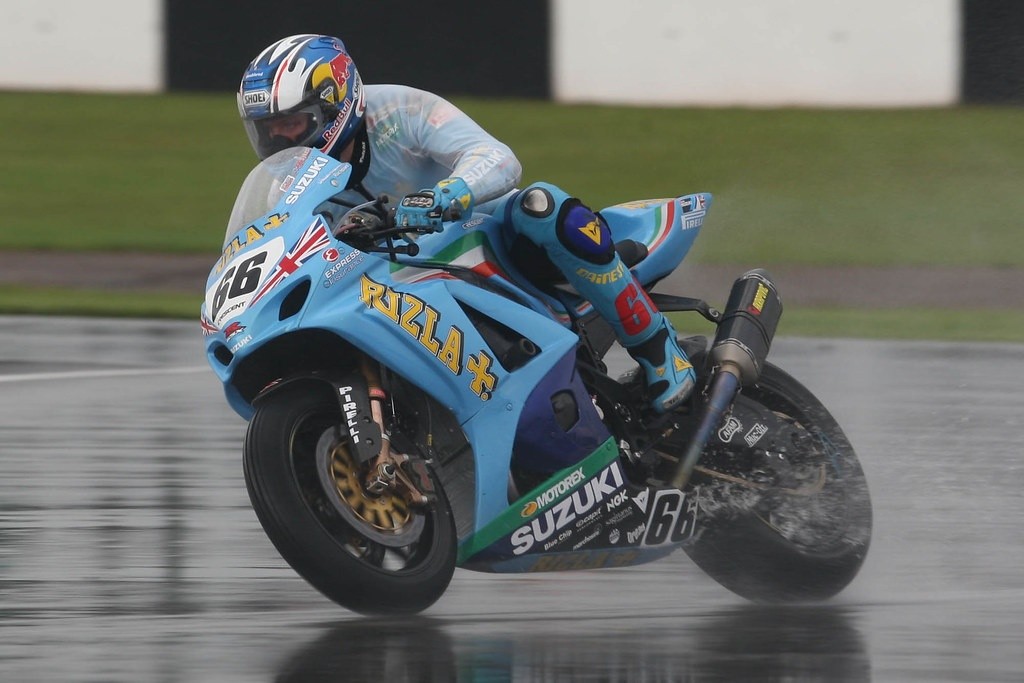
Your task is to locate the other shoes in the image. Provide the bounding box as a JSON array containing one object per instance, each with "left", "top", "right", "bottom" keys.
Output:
[{"left": 636, "top": 320, "right": 697, "bottom": 412}]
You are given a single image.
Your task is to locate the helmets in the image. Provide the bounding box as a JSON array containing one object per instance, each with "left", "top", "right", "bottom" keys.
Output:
[{"left": 235, "top": 33, "right": 368, "bottom": 156}]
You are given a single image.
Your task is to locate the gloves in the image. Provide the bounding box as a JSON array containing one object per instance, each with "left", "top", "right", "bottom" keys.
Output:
[{"left": 395, "top": 175, "right": 478, "bottom": 238}]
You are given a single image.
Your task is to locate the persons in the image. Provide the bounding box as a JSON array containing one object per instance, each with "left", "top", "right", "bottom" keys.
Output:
[{"left": 235, "top": 33, "right": 696, "bottom": 414}]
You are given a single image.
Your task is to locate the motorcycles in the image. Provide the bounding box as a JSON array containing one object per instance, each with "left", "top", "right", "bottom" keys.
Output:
[{"left": 200, "top": 146, "right": 873, "bottom": 616}]
